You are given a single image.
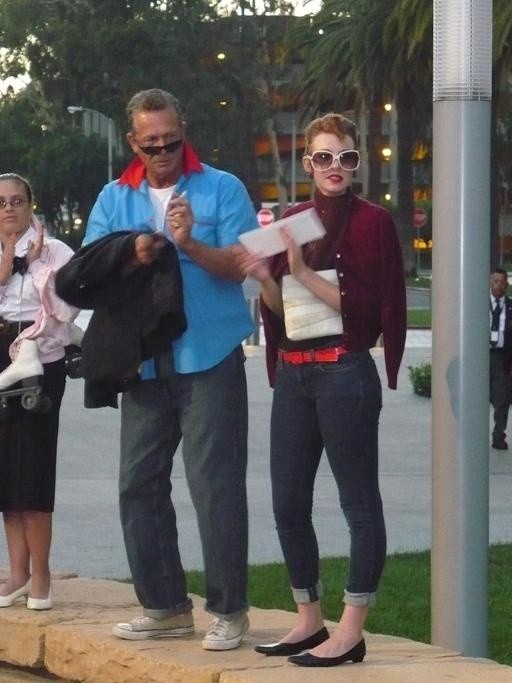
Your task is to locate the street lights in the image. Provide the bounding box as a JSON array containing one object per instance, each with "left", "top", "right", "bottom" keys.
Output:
[{"left": 65, "top": 104, "right": 116, "bottom": 182}]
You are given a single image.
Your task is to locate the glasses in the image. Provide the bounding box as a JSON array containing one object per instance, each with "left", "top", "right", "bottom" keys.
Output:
[
  {"left": 301, "top": 148, "right": 362, "bottom": 174},
  {"left": 0, "top": 197, "right": 32, "bottom": 207},
  {"left": 132, "top": 138, "right": 184, "bottom": 154}
]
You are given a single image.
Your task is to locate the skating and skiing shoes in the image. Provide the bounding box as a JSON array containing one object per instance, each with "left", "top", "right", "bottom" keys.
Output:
[{"left": 0, "top": 337, "right": 46, "bottom": 411}]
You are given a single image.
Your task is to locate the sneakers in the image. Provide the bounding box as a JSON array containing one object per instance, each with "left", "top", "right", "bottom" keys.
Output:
[
  {"left": 490, "top": 430, "right": 508, "bottom": 452},
  {"left": 200, "top": 613, "right": 251, "bottom": 650},
  {"left": 110, "top": 608, "right": 196, "bottom": 641}
]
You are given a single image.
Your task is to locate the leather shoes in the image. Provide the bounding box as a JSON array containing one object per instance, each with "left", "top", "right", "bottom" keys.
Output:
[
  {"left": 286, "top": 636, "right": 369, "bottom": 667},
  {"left": 252, "top": 624, "right": 331, "bottom": 658}
]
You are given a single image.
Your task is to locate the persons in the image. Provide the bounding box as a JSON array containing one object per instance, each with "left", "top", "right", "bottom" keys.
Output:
[
  {"left": 0, "top": 170, "right": 91, "bottom": 613},
  {"left": 231, "top": 108, "right": 411, "bottom": 666},
  {"left": 488, "top": 265, "right": 511, "bottom": 451},
  {"left": 80, "top": 86, "right": 268, "bottom": 653}
]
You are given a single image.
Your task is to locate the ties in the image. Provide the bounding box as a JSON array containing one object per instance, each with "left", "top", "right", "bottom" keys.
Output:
[{"left": 489, "top": 295, "right": 501, "bottom": 350}]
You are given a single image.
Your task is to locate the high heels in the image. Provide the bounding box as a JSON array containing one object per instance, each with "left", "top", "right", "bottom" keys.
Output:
[
  {"left": 0, "top": 574, "right": 35, "bottom": 607},
  {"left": 26, "top": 578, "right": 54, "bottom": 611}
]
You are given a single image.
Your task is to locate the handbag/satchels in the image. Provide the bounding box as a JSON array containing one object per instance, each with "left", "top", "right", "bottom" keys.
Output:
[{"left": 279, "top": 265, "right": 346, "bottom": 340}]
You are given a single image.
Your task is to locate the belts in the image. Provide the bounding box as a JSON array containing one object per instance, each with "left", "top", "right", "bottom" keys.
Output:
[{"left": 274, "top": 344, "right": 353, "bottom": 367}]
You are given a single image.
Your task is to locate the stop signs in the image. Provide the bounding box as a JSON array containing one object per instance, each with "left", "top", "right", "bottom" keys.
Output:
[
  {"left": 413, "top": 207, "right": 428, "bottom": 228},
  {"left": 254, "top": 208, "right": 275, "bottom": 227}
]
